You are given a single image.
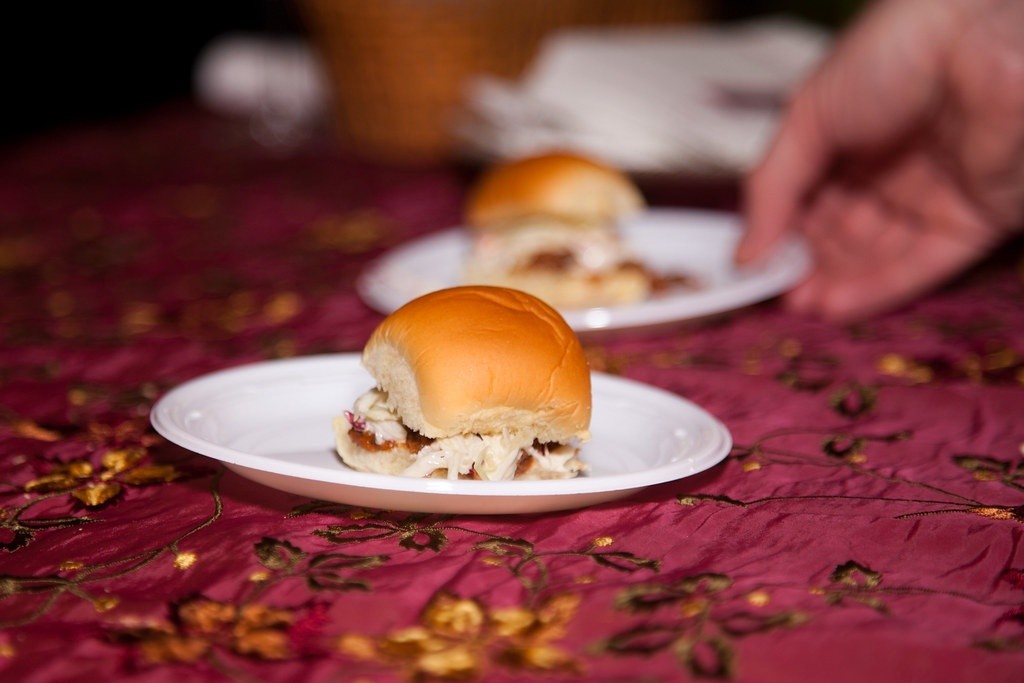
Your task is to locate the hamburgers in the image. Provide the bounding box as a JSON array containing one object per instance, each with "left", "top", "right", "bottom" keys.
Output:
[
  {"left": 465, "top": 150, "right": 653, "bottom": 307},
  {"left": 335, "top": 284, "right": 590, "bottom": 481}
]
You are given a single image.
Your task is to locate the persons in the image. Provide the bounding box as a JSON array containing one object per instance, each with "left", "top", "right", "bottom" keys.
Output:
[{"left": 733, "top": 0, "right": 1024, "bottom": 325}]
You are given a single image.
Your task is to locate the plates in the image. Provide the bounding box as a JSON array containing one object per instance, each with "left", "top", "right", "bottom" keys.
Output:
[
  {"left": 151, "top": 352, "right": 735, "bottom": 515},
  {"left": 356, "top": 205, "right": 816, "bottom": 346}
]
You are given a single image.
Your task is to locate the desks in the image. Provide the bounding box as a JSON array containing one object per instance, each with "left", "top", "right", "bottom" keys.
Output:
[{"left": 0, "top": 119, "right": 1024, "bottom": 682}]
